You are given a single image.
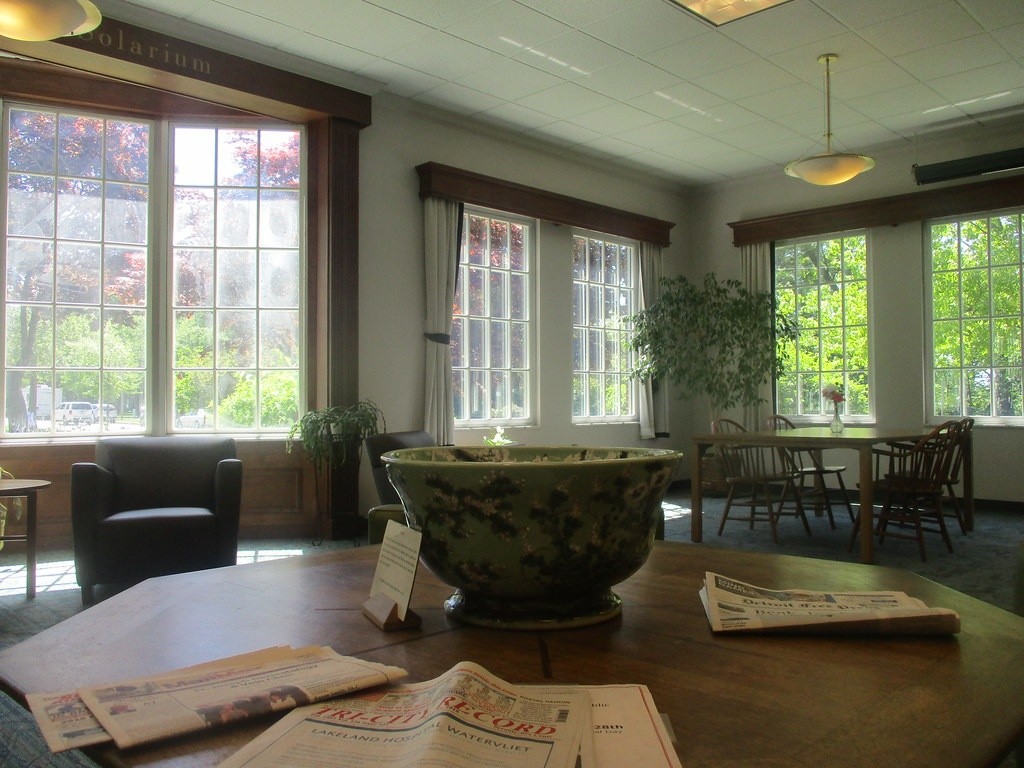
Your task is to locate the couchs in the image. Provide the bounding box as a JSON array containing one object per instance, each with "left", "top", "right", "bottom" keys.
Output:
[
  {"left": 72, "top": 436, "right": 243, "bottom": 599},
  {"left": 365, "top": 429, "right": 440, "bottom": 542}
]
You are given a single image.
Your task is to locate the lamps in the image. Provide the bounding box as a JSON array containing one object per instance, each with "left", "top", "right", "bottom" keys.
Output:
[
  {"left": 782, "top": 53, "right": 876, "bottom": 186},
  {"left": 1, "top": 1, "right": 102, "bottom": 42}
]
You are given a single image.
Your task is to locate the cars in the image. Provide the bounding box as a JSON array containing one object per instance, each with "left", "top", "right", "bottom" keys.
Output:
[
  {"left": 91, "top": 404, "right": 117, "bottom": 423},
  {"left": 176, "top": 406, "right": 210, "bottom": 428}
]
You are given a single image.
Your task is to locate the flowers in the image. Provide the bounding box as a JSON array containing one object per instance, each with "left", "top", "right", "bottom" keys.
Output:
[{"left": 821, "top": 382, "right": 853, "bottom": 414}]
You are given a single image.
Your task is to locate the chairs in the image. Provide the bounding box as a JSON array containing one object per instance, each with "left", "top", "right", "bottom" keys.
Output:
[
  {"left": 711, "top": 414, "right": 854, "bottom": 541},
  {"left": 849, "top": 417, "right": 980, "bottom": 562}
]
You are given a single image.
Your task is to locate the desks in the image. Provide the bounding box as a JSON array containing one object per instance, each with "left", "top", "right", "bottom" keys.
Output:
[
  {"left": 0, "top": 477, "right": 51, "bottom": 606},
  {"left": 0, "top": 539, "right": 1024, "bottom": 768},
  {"left": 691, "top": 427, "right": 975, "bottom": 559}
]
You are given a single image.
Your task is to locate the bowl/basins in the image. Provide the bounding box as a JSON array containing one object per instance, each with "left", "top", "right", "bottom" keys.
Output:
[{"left": 378, "top": 443, "right": 682, "bottom": 630}]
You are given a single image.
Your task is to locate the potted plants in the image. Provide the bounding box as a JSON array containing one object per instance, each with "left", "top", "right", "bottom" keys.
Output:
[
  {"left": 619, "top": 270, "right": 803, "bottom": 498},
  {"left": 286, "top": 399, "right": 386, "bottom": 470}
]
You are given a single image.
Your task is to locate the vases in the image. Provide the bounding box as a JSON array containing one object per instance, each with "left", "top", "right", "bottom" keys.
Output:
[{"left": 829, "top": 413, "right": 844, "bottom": 433}]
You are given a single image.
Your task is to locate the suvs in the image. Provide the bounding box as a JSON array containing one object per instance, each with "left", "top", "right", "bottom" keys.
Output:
[{"left": 50, "top": 401, "right": 94, "bottom": 426}]
